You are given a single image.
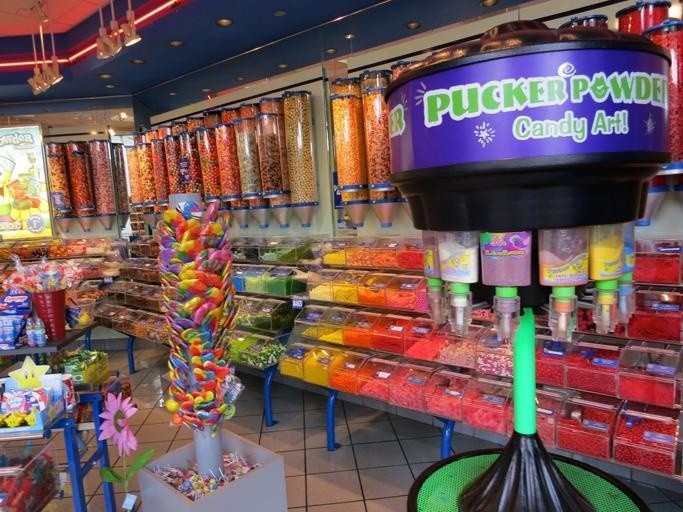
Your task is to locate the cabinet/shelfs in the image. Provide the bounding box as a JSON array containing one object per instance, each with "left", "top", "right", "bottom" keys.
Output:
[
  {"left": 1, "top": 239, "right": 114, "bottom": 352},
  {"left": 1, "top": 319, "right": 125, "bottom": 511}
]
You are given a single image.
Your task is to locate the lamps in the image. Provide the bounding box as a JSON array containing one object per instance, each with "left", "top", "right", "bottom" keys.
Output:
[
  {"left": 92, "top": 0, "right": 144, "bottom": 61},
  {"left": 26, "top": 1, "right": 66, "bottom": 97}
]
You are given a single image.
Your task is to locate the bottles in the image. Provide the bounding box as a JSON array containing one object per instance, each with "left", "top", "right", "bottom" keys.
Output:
[{"left": 25, "top": 317, "right": 45, "bottom": 347}]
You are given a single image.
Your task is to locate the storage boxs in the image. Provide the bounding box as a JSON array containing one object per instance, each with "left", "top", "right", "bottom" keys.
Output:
[{"left": 135, "top": 426, "right": 289, "bottom": 511}]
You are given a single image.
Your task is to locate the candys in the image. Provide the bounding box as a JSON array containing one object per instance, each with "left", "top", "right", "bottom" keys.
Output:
[
  {"left": 151, "top": 448, "right": 261, "bottom": 501},
  {"left": 156, "top": 203, "right": 242, "bottom": 437},
  {"left": 279, "top": 238, "right": 683, "bottom": 473},
  {"left": 94, "top": 238, "right": 313, "bottom": 371},
  {"left": 643, "top": 19, "right": 683, "bottom": 165},
  {"left": 47, "top": 67, "right": 393, "bottom": 232}
]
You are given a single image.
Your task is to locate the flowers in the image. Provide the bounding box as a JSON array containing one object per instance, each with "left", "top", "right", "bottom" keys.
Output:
[{"left": 97, "top": 392, "right": 156, "bottom": 510}]
